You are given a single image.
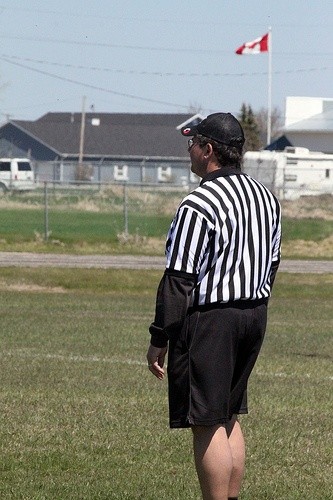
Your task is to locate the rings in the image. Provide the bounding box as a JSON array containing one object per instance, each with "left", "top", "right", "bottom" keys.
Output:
[{"left": 147, "top": 364, "right": 152, "bottom": 367}]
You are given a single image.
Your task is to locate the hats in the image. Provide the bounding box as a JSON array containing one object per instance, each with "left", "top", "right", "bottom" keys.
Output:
[{"left": 180, "top": 112, "right": 245, "bottom": 148}]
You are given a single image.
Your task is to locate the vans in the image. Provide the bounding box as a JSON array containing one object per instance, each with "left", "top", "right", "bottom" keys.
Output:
[{"left": 0, "top": 157, "right": 36, "bottom": 199}]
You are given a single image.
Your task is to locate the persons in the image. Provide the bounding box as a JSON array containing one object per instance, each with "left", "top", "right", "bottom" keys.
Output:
[{"left": 146, "top": 113, "right": 281, "bottom": 500}]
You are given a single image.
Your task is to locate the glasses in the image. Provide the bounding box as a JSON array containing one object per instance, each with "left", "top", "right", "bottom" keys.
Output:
[{"left": 188, "top": 139, "right": 208, "bottom": 148}]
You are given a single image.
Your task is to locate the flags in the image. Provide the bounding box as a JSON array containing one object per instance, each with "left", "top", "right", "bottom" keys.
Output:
[{"left": 235, "top": 31, "right": 270, "bottom": 54}]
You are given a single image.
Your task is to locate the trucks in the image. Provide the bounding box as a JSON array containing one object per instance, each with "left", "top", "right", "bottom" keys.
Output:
[{"left": 242, "top": 145, "right": 333, "bottom": 201}]
